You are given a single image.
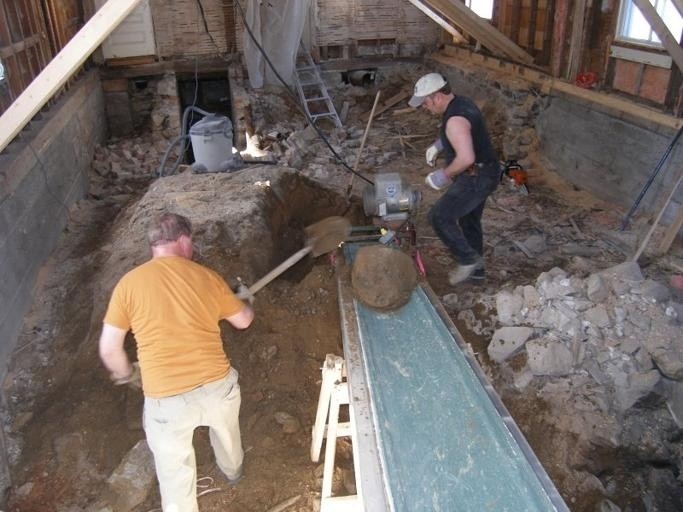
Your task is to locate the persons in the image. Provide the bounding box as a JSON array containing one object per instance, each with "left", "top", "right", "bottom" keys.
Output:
[
  {"left": 99, "top": 212, "right": 258, "bottom": 512},
  {"left": 408, "top": 74, "right": 502, "bottom": 287}
]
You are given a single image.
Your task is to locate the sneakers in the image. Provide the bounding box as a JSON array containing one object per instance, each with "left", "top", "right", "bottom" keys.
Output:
[
  {"left": 450, "top": 258, "right": 484, "bottom": 284},
  {"left": 227, "top": 469, "right": 243, "bottom": 483}
]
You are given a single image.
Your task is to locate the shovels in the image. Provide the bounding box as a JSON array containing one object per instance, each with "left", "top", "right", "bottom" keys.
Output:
[
  {"left": 347, "top": 90, "right": 381, "bottom": 200},
  {"left": 248, "top": 216, "right": 352, "bottom": 295}
]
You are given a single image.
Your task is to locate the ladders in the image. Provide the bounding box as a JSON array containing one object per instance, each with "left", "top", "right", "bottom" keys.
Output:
[{"left": 293, "top": 38, "right": 342, "bottom": 129}]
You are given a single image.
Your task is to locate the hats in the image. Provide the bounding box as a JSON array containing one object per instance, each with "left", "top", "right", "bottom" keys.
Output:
[{"left": 408, "top": 72, "right": 447, "bottom": 107}]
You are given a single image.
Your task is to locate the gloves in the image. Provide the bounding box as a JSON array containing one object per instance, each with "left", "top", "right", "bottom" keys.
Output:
[
  {"left": 232, "top": 283, "right": 255, "bottom": 307},
  {"left": 424, "top": 169, "right": 452, "bottom": 190},
  {"left": 426, "top": 139, "right": 443, "bottom": 166},
  {"left": 110, "top": 362, "right": 142, "bottom": 390}
]
